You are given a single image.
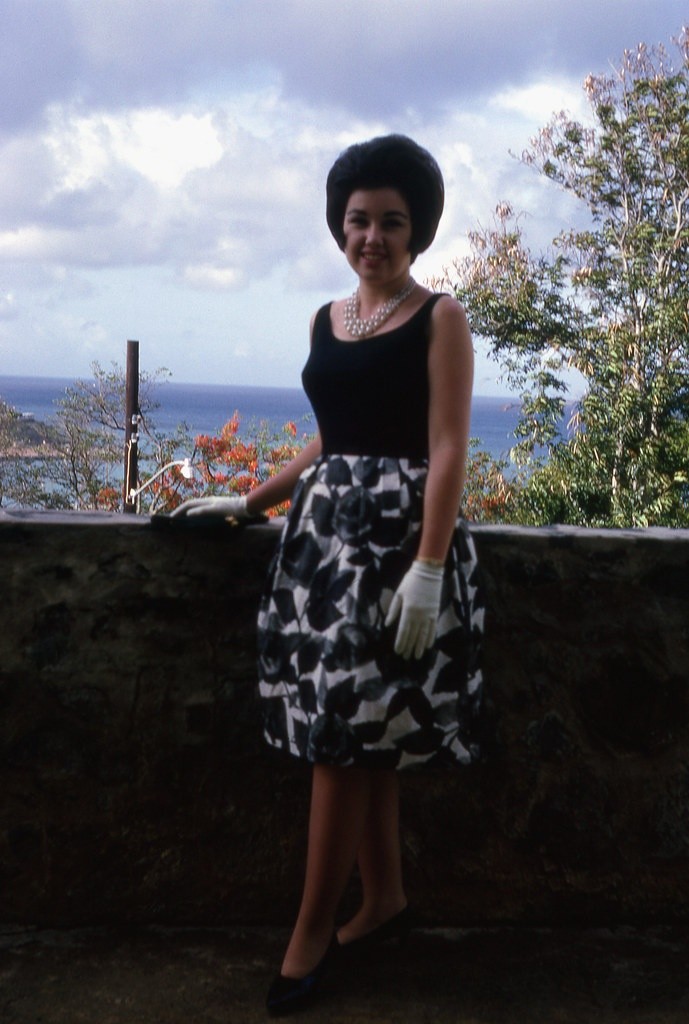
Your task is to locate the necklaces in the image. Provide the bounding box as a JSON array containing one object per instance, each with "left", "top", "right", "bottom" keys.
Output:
[{"left": 343, "top": 275, "right": 416, "bottom": 337}]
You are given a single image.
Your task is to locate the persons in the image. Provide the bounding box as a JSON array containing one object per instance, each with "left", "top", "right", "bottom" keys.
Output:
[{"left": 169, "top": 134, "right": 474, "bottom": 1016}]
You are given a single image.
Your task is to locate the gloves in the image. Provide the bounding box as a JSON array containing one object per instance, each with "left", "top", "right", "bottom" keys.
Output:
[
  {"left": 384, "top": 561, "right": 444, "bottom": 659},
  {"left": 166, "top": 497, "right": 254, "bottom": 520}
]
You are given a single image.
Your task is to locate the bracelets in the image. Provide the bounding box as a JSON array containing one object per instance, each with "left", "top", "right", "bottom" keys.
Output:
[{"left": 416, "top": 555, "right": 445, "bottom": 566}]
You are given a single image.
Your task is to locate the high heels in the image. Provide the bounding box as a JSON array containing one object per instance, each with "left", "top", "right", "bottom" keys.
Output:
[
  {"left": 266, "top": 930, "right": 339, "bottom": 1011},
  {"left": 334, "top": 901, "right": 414, "bottom": 959}
]
